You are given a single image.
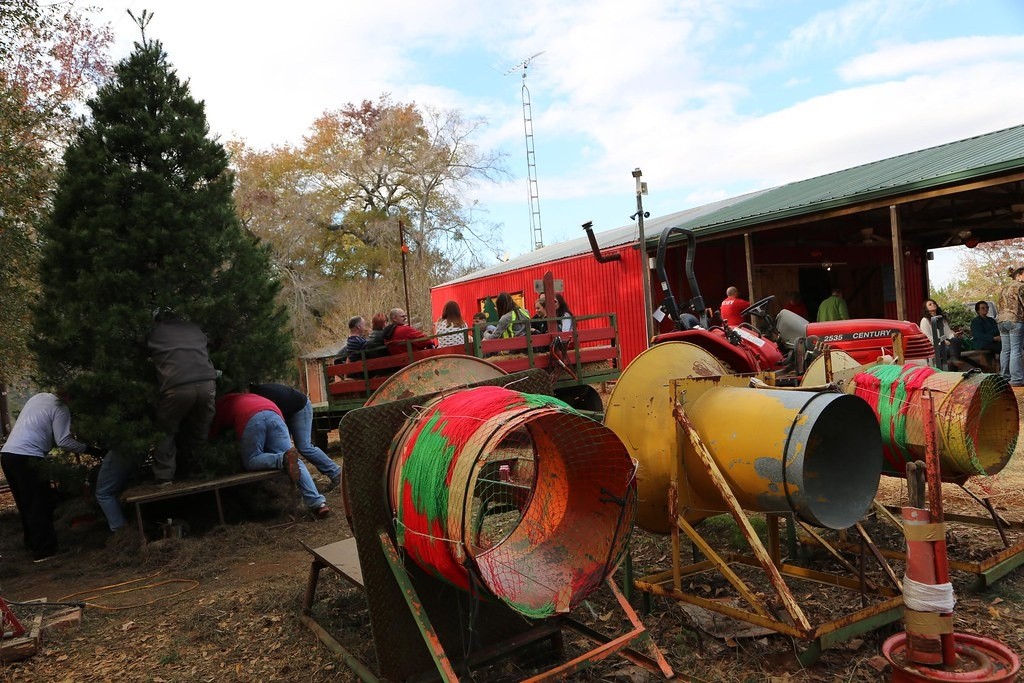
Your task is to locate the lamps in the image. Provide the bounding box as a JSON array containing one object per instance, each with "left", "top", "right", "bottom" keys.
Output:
[
  {"left": 927, "top": 252, "right": 934, "bottom": 261},
  {"left": 821, "top": 262, "right": 833, "bottom": 271},
  {"left": 904, "top": 247, "right": 911, "bottom": 257}
]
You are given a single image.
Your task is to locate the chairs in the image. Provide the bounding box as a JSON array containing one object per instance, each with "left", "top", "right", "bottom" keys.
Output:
[{"left": 681, "top": 313, "right": 703, "bottom": 331}]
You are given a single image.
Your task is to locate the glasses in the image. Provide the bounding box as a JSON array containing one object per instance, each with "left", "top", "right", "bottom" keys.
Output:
[
  {"left": 400, "top": 315, "right": 407, "bottom": 318},
  {"left": 554, "top": 300, "right": 557, "bottom": 303},
  {"left": 979, "top": 306, "right": 989, "bottom": 309}
]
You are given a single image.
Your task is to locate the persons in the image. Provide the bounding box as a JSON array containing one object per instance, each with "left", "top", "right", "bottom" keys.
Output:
[
  {"left": 209, "top": 392, "right": 330, "bottom": 513},
  {"left": 432, "top": 301, "right": 468, "bottom": 349},
  {"left": 95, "top": 446, "right": 189, "bottom": 548},
  {"left": 147, "top": 312, "right": 216, "bottom": 487},
  {"left": 240, "top": 383, "right": 340, "bottom": 492},
  {"left": 0, "top": 384, "right": 101, "bottom": 563},
  {"left": 971, "top": 301, "right": 1010, "bottom": 374},
  {"left": 333, "top": 308, "right": 433, "bottom": 378},
  {"left": 720, "top": 286, "right": 751, "bottom": 327},
  {"left": 816, "top": 285, "right": 849, "bottom": 322},
  {"left": 783, "top": 291, "right": 807, "bottom": 320},
  {"left": 472, "top": 292, "right": 576, "bottom": 372},
  {"left": 919, "top": 299, "right": 962, "bottom": 372},
  {"left": 1000, "top": 267, "right": 1024, "bottom": 387}
]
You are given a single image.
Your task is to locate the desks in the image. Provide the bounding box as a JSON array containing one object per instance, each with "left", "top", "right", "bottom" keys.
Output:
[{"left": 125, "top": 469, "right": 299, "bottom": 548}]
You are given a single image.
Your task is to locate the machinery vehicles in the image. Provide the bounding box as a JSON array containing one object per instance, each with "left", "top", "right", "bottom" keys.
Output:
[{"left": 648, "top": 224, "right": 936, "bottom": 394}]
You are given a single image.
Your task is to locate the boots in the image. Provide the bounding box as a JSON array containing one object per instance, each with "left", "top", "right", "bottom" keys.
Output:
[{"left": 946, "top": 346, "right": 964, "bottom": 367}]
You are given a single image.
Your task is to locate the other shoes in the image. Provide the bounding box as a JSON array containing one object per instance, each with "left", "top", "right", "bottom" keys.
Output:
[
  {"left": 190, "top": 467, "right": 205, "bottom": 477},
  {"left": 316, "top": 503, "right": 329, "bottom": 514},
  {"left": 155, "top": 478, "right": 172, "bottom": 488},
  {"left": 32, "top": 547, "right": 70, "bottom": 562},
  {"left": 283, "top": 447, "right": 301, "bottom": 483},
  {"left": 321, "top": 475, "right": 341, "bottom": 494}
]
